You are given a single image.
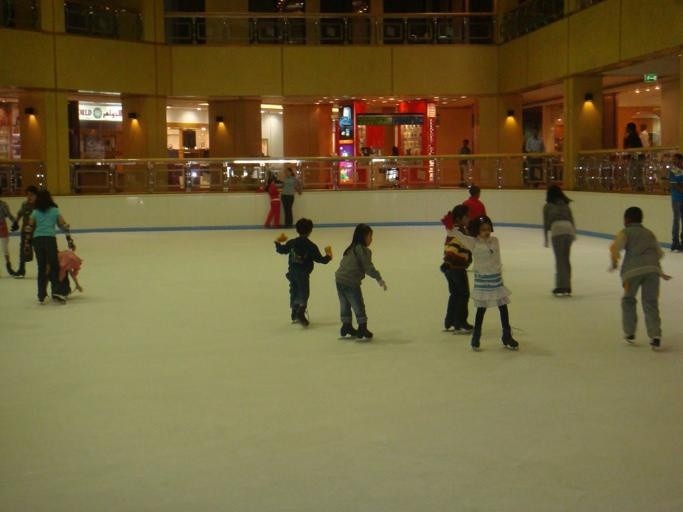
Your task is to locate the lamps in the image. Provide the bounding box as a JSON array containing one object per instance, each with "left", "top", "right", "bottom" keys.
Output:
[
  {"left": 215, "top": 116, "right": 223, "bottom": 122},
  {"left": 23, "top": 106, "right": 34, "bottom": 115},
  {"left": 126, "top": 112, "right": 136, "bottom": 119},
  {"left": 584, "top": 92, "right": 593, "bottom": 102},
  {"left": 507, "top": 109, "right": 514, "bottom": 117}
]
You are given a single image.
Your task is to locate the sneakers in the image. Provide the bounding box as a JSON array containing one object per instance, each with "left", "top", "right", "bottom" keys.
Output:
[
  {"left": 341, "top": 328, "right": 373, "bottom": 338},
  {"left": 446, "top": 319, "right": 473, "bottom": 331},
  {"left": 650, "top": 337, "right": 660, "bottom": 346}
]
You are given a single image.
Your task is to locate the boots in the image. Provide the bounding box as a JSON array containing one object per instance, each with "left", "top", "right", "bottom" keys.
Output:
[
  {"left": 472, "top": 326, "right": 481, "bottom": 347},
  {"left": 292, "top": 303, "right": 309, "bottom": 325},
  {"left": 502, "top": 326, "right": 518, "bottom": 347}
]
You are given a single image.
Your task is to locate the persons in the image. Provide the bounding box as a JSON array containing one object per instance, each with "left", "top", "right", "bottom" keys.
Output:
[
  {"left": 607, "top": 207, "right": 673, "bottom": 348},
  {"left": 334, "top": 223, "right": 388, "bottom": 339},
  {"left": 440, "top": 205, "right": 474, "bottom": 331},
  {"left": 263, "top": 177, "right": 282, "bottom": 229},
  {"left": 525, "top": 127, "right": 544, "bottom": 187},
  {"left": 668, "top": 153, "right": 683, "bottom": 252},
  {"left": 22, "top": 188, "right": 79, "bottom": 305},
  {"left": 457, "top": 139, "right": 471, "bottom": 187},
  {"left": 637, "top": 124, "right": 650, "bottom": 157},
  {"left": 281, "top": 167, "right": 302, "bottom": 228},
  {"left": 542, "top": 186, "right": 578, "bottom": 293},
  {"left": 441, "top": 210, "right": 518, "bottom": 348},
  {"left": 11, "top": 185, "right": 38, "bottom": 276},
  {"left": 0, "top": 200, "right": 15, "bottom": 277},
  {"left": 462, "top": 186, "right": 487, "bottom": 221},
  {"left": 623, "top": 123, "right": 646, "bottom": 192},
  {"left": 274, "top": 218, "right": 333, "bottom": 327}
]
